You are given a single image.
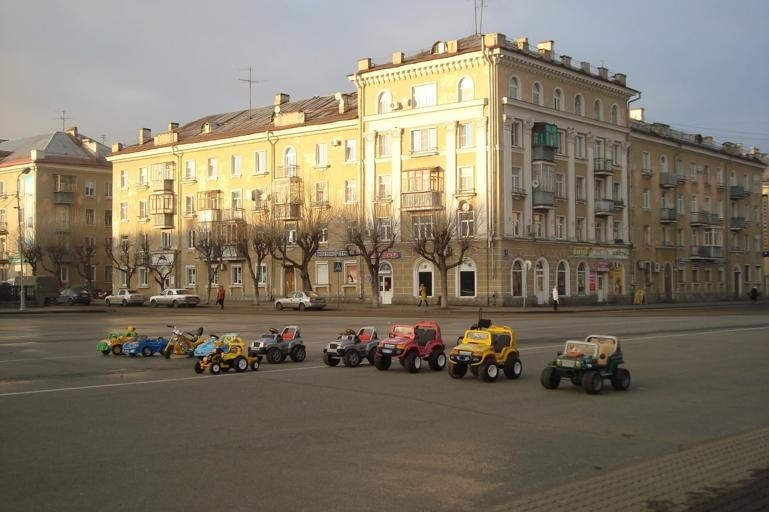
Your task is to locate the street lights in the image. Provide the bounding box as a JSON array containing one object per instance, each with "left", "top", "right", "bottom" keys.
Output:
[{"left": 16, "top": 167, "right": 32, "bottom": 311}]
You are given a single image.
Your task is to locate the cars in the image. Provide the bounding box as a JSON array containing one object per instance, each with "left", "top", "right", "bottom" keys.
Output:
[
  {"left": 274, "top": 290, "right": 326, "bottom": 311},
  {"left": 98, "top": 288, "right": 112, "bottom": 298},
  {"left": 56, "top": 288, "right": 91, "bottom": 305},
  {"left": 150, "top": 289, "right": 200, "bottom": 307},
  {"left": 104, "top": 289, "right": 145, "bottom": 306}
]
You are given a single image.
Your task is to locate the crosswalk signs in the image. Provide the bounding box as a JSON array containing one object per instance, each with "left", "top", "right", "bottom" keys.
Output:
[{"left": 333, "top": 262, "right": 342, "bottom": 272}]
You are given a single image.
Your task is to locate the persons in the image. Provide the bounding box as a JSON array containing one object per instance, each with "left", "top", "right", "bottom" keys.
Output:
[
  {"left": 751, "top": 285, "right": 759, "bottom": 301},
  {"left": 551, "top": 284, "right": 560, "bottom": 311},
  {"left": 417, "top": 283, "right": 431, "bottom": 308},
  {"left": 215, "top": 285, "right": 227, "bottom": 311}
]
